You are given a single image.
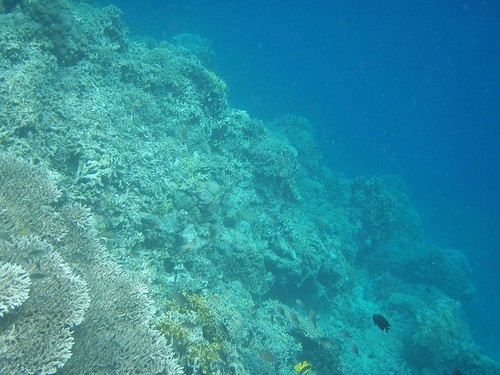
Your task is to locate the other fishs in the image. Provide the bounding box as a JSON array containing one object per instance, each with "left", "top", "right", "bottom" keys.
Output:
[
  {"left": 373, "top": 313, "right": 392, "bottom": 334},
  {"left": 250, "top": 304, "right": 377, "bottom": 375}
]
